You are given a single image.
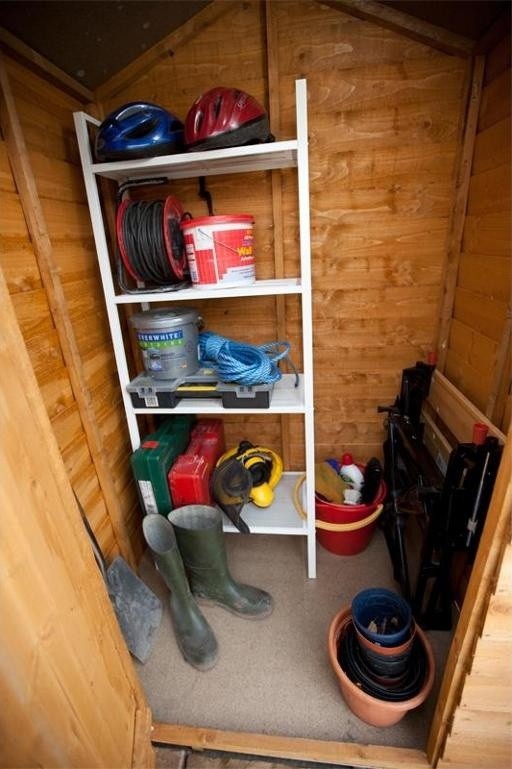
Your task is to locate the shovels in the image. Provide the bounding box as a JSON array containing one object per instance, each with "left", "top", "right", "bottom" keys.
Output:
[{"left": 71, "top": 486, "right": 165, "bottom": 666}]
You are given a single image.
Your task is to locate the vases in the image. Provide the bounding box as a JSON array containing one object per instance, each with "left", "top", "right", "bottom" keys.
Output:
[{"left": 330, "top": 587, "right": 437, "bottom": 728}]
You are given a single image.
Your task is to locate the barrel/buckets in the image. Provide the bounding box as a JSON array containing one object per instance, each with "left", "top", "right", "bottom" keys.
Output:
[
  {"left": 130, "top": 307, "right": 203, "bottom": 383},
  {"left": 182, "top": 213, "right": 257, "bottom": 288},
  {"left": 295, "top": 452, "right": 389, "bottom": 558}
]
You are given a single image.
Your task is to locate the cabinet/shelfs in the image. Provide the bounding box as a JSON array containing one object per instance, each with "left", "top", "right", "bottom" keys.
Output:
[{"left": 73, "top": 77, "right": 319, "bottom": 583}]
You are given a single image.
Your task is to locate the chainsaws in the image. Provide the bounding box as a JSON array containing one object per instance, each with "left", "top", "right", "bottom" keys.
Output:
[{"left": 216, "top": 439, "right": 284, "bottom": 535}]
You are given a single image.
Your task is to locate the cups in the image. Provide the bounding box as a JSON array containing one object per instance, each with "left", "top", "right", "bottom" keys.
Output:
[{"left": 472, "top": 422, "right": 489, "bottom": 446}]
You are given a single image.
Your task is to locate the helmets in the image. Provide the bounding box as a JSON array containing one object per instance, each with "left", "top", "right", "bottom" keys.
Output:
[
  {"left": 94, "top": 102, "right": 181, "bottom": 161},
  {"left": 184, "top": 89, "right": 271, "bottom": 151}
]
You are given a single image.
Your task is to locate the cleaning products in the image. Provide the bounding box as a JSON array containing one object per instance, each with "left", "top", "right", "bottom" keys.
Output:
[{"left": 337, "top": 454, "right": 365, "bottom": 492}]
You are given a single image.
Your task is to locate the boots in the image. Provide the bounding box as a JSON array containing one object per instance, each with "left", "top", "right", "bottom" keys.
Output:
[
  {"left": 143, "top": 514, "right": 219, "bottom": 672},
  {"left": 168, "top": 506, "right": 274, "bottom": 620}
]
